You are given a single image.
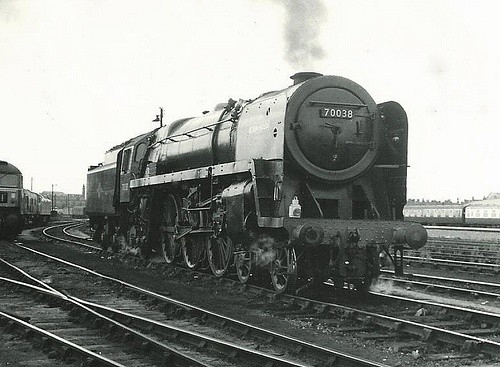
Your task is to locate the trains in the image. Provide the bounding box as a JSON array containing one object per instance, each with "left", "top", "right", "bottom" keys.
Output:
[
  {"left": 83, "top": 71, "right": 429, "bottom": 296},
  {"left": 0, "top": 160, "right": 51, "bottom": 242}
]
